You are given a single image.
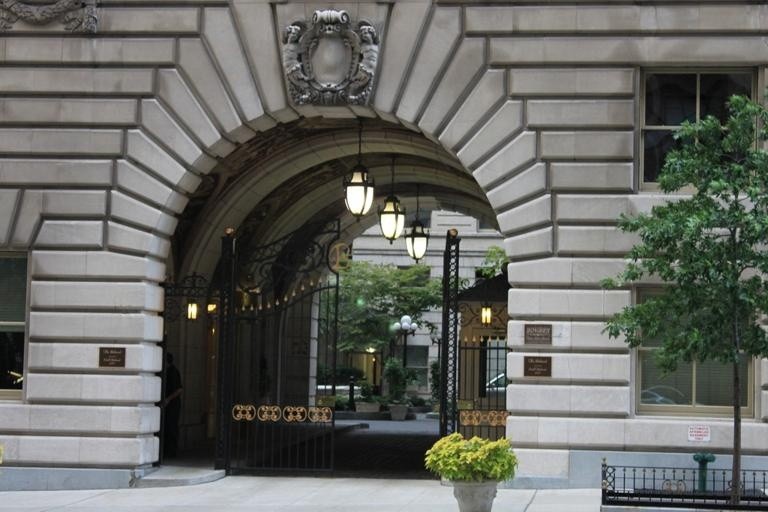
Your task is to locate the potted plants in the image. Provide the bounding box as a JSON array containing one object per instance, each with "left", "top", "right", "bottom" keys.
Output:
[
  {"left": 424, "top": 432, "right": 518, "bottom": 512},
  {"left": 354, "top": 381, "right": 380, "bottom": 413},
  {"left": 383, "top": 356, "right": 410, "bottom": 420}
]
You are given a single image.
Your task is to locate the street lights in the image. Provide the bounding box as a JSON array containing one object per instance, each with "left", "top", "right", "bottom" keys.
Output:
[{"left": 389, "top": 315, "right": 418, "bottom": 367}]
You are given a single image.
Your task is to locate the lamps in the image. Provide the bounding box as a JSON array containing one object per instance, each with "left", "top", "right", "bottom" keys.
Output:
[
  {"left": 481, "top": 301, "right": 492, "bottom": 327},
  {"left": 343, "top": 115, "right": 374, "bottom": 222},
  {"left": 407, "top": 183, "right": 429, "bottom": 263},
  {"left": 186, "top": 297, "right": 198, "bottom": 320},
  {"left": 377, "top": 152, "right": 406, "bottom": 243}
]
